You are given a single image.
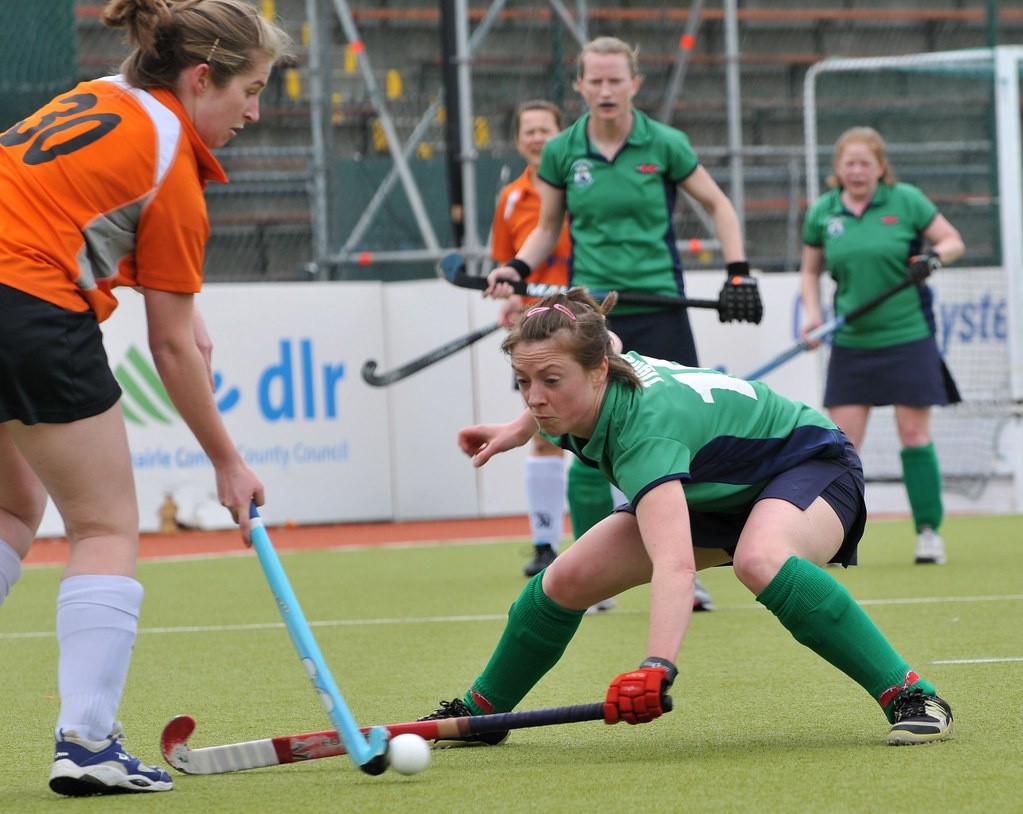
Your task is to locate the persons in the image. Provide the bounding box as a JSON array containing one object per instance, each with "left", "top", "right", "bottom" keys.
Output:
[
  {"left": 799, "top": 126, "right": 965, "bottom": 563},
  {"left": 418, "top": 288, "right": 952, "bottom": 746},
  {"left": 0, "top": 0, "right": 285, "bottom": 797},
  {"left": 480, "top": 36, "right": 763, "bottom": 612}
]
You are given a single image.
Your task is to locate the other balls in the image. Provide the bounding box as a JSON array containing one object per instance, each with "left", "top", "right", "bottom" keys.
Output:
[{"left": 388, "top": 732, "right": 432, "bottom": 777}]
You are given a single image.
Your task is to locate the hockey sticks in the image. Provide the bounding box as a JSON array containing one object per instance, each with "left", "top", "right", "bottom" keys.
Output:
[
  {"left": 438, "top": 250, "right": 719, "bottom": 309},
  {"left": 360, "top": 321, "right": 502, "bottom": 389},
  {"left": 247, "top": 497, "right": 393, "bottom": 778},
  {"left": 159, "top": 693, "right": 675, "bottom": 777},
  {"left": 716, "top": 257, "right": 942, "bottom": 383}
]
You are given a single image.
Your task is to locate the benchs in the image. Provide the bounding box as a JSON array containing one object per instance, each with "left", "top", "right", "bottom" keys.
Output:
[{"left": 75, "top": 7, "right": 1023, "bottom": 277}]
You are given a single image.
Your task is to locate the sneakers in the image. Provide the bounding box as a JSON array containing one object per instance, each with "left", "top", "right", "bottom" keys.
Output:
[
  {"left": 49, "top": 721, "right": 173, "bottom": 797},
  {"left": 416, "top": 698, "right": 509, "bottom": 748},
  {"left": 915, "top": 527, "right": 945, "bottom": 562},
  {"left": 884, "top": 689, "right": 954, "bottom": 745},
  {"left": 525, "top": 548, "right": 557, "bottom": 577},
  {"left": 692, "top": 580, "right": 713, "bottom": 612}
]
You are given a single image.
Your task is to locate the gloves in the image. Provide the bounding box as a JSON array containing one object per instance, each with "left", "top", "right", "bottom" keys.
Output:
[
  {"left": 718, "top": 275, "right": 763, "bottom": 326},
  {"left": 909, "top": 252, "right": 940, "bottom": 282},
  {"left": 604, "top": 656, "right": 679, "bottom": 725}
]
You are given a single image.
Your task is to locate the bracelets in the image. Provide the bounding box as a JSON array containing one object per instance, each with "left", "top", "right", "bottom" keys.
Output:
[{"left": 506, "top": 259, "right": 531, "bottom": 279}]
